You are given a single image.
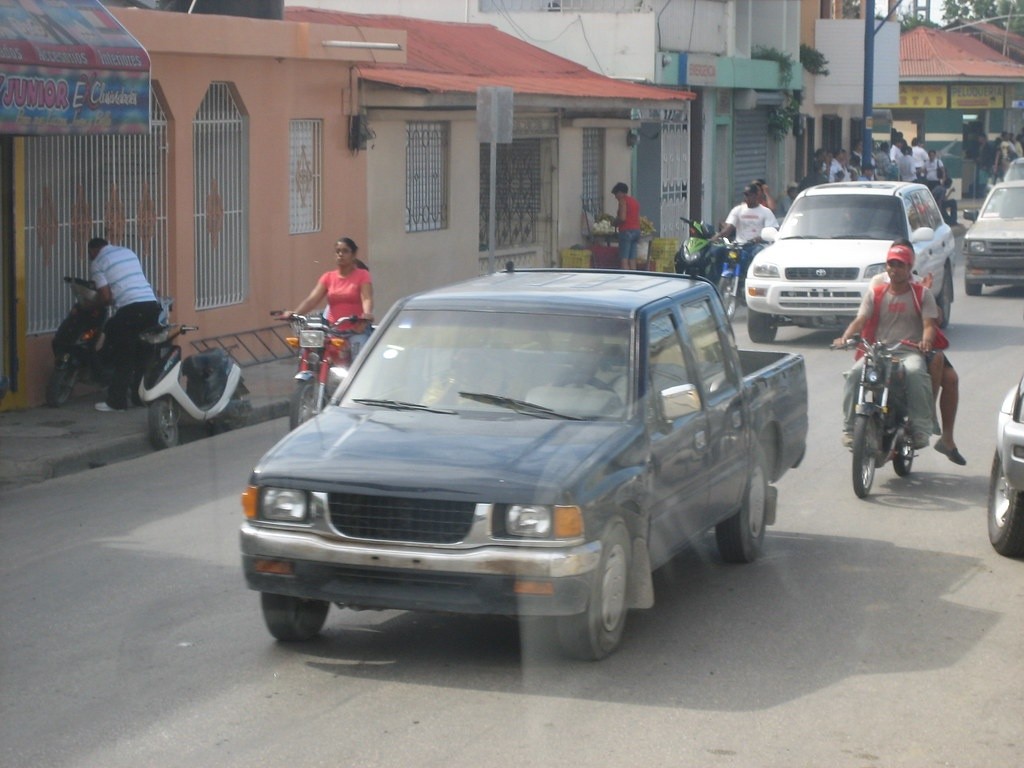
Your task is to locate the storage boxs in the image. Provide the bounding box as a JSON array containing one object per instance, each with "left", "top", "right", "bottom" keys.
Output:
[
  {"left": 589, "top": 247, "right": 618, "bottom": 268},
  {"left": 562, "top": 250, "right": 592, "bottom": 269}
]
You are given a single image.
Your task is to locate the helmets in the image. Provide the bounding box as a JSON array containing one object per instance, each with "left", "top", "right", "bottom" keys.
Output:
[{"left": 742, "top": 184, "right": 760, "bottom": 195}]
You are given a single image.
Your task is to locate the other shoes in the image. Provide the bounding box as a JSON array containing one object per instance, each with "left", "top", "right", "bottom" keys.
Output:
[
  {"left": 842, "top": 435, "right": 854, "bottom": 446},
  {"left": 913, "top": 431, "right": 929, "bottom": 448},
  {"left": 935, "top": 439, "right": 966, "bottom": 465},
  {"left": 933, "top": 422, "right": 941, "bottom": 436},
  {"left": 94, "top": 401, "right": 125, "bottom": 412}
]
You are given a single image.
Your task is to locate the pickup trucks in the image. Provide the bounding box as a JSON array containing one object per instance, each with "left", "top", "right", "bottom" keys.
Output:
[{"left": 238, "top": 265, "right": 809, "bottom": 663}]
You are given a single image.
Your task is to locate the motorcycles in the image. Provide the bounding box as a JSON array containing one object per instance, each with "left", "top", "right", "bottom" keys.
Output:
[
  {"left": 828, "top": 335, "right": 935, "bottom": 497},
  {"left": 710, "top": 233, "right": 757, "bottom": 323},
  {"left": 675, "top": 217, "right": 725, "bottom": 285},
  {"left": 271, "top": 310, "right": 409, "bottom": 431}
]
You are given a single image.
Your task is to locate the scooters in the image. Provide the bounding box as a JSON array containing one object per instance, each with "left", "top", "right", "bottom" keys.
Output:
[
  {"left": 44, "top": 277, "right": 174, "bottom": 408},
  {"left": 135, "top": 324, "right": 255, "bottom": 450}
]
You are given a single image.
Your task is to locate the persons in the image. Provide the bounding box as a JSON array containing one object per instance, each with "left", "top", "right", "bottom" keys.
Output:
[
  {"left": 273, "top": 236, "right": 373, "bottom": 362},
  {"left": 87, "top": 238, "right": 163, "bottom": 412},
  {"left": 832, "top": 239, "right": 965, "bottom": 466},
  {"left": 963, "top": 120, "right": 1024, "bottom": 185},
  {"left": 610, "top": 183, "right": 640, "bottom": 270},
  {"left": 753, "top": 179, "right": 778, "bottom": 214},
  {"left": 784, "top": 127, "right": 958, "bottom": 226},
  {"left": 710, "top": 183, "right": 780, "bottom": 307}
]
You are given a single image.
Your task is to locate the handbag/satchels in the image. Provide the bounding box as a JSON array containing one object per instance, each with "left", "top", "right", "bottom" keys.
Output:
[{"left": 937, "top": 161, "right": 947, "bottom": 179}]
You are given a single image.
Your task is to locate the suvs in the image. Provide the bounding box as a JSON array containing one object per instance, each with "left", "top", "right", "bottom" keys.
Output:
[{"left": 745, "top": 181, "right": 956, "bottom": 344}]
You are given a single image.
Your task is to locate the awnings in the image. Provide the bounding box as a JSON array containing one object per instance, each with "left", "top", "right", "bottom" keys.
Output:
[{"left": 0, "top": 0, "right": 152, "bottom": 134}]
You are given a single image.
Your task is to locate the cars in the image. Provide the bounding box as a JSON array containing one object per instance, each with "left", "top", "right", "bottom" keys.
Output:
[
  {"left": 988, "top": 158, "right": 1024, "bottom": 211},
  {"left": 987, "top": 374, "right": 1024, "bottom": 552},
  {"left": 963, "top": 181, "right": 1024, "bottom": 295}
]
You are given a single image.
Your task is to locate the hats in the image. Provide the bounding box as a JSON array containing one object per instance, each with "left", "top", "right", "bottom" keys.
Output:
[{"left": 886, "top": 245, "right": 913, "bottom": 264}]
